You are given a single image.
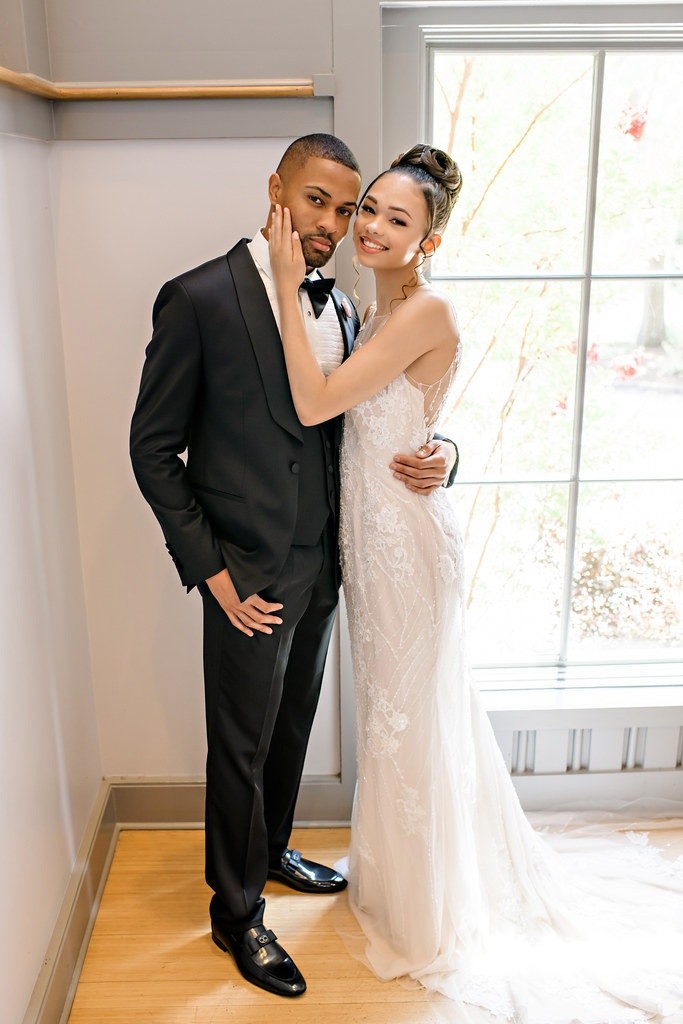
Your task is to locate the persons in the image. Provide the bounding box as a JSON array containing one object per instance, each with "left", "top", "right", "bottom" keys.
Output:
[
  {"left": 130, "top": 132, "right": 460, "bottom": 999},
  {"left": 267, "top": 144, "right": 683, "bottom": 1024}
]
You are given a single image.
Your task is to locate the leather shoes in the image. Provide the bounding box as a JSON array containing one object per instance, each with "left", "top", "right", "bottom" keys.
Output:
[
  {"left": 211, "top": 920, "right": 307, "bottom": 998},
  {"left": 266, "top": 846, "right": 348, "bottom": 894}
]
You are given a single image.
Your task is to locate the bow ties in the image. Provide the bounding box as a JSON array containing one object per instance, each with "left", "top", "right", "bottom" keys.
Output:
[{"left": 299, "top": 277, "right": 337, "bottom": 319}]
